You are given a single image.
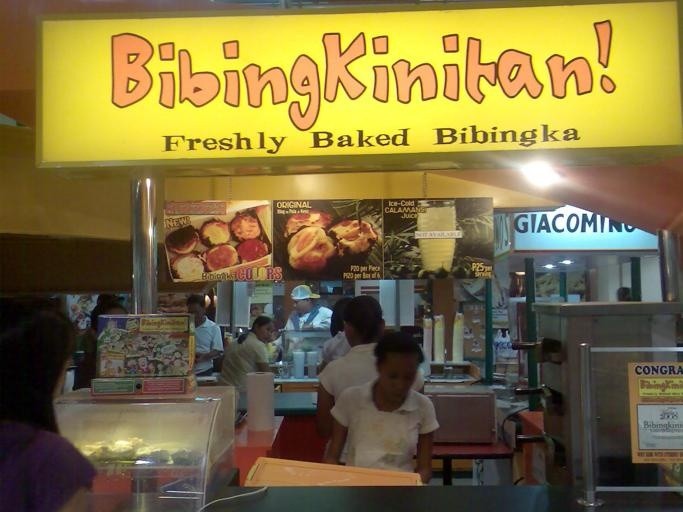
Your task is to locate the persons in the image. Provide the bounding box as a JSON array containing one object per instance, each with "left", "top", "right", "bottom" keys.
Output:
[
  {"left": 97, "top": 293, "right": 126, "bottom": 304},
  {"left": 185, "top": 294, "right": 225, "bottom": 376},
  {"left": 321, "top": 298, "right": 351, "bottom": 370},
  {"left": 220, "top": 316, "right": 275, "bottom": 387},
  {"left": 316, "top": 295, "right": 423, "bottom": 465},
  {"left": 72, "top": 305, "right": 128, "bottom": 390},
  {"left": 330, "top": 332, "right": 440, "bottom": 484},
  {"left": 271, "top": 284, "right": 334, "bottom": 350},
  {"left": 0, "top": 295, "right": 98, "bottom": 512}
]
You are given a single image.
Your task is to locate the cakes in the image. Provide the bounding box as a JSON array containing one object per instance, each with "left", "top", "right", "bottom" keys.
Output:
[{"left": 283, "top": 210, "right": 377, "bottom": 274}]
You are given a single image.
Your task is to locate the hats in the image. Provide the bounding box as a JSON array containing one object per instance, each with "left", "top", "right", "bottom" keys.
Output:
[{"left": 290, "top": 285, "right": 321, "bottom": 300}]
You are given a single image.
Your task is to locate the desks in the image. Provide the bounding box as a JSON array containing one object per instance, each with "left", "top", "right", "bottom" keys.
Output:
[{"left": 413, "top": 442, "right": 514, "bottom": 485}]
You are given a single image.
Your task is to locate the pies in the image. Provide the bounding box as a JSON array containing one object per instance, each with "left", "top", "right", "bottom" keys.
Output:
[{"left": 166, "top": 212, "right": 269, "bottom": 279}]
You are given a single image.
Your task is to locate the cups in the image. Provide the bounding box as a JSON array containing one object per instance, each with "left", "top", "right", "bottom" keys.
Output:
[
  {"left": 293, "top": 351, "right": 319, "bottom": 379},
  {"left": 433, "top": 313, "right": 464, "bottom": 365}
]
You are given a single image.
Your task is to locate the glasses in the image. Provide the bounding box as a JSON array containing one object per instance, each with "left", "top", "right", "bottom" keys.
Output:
[{"left": 70, "top": 350, "right": 85, "bottom": 365}]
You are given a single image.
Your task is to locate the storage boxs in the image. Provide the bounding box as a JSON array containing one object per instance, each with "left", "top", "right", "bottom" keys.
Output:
[{"left": 424, "top": 385, "right": 498, "bottom": 444}]
